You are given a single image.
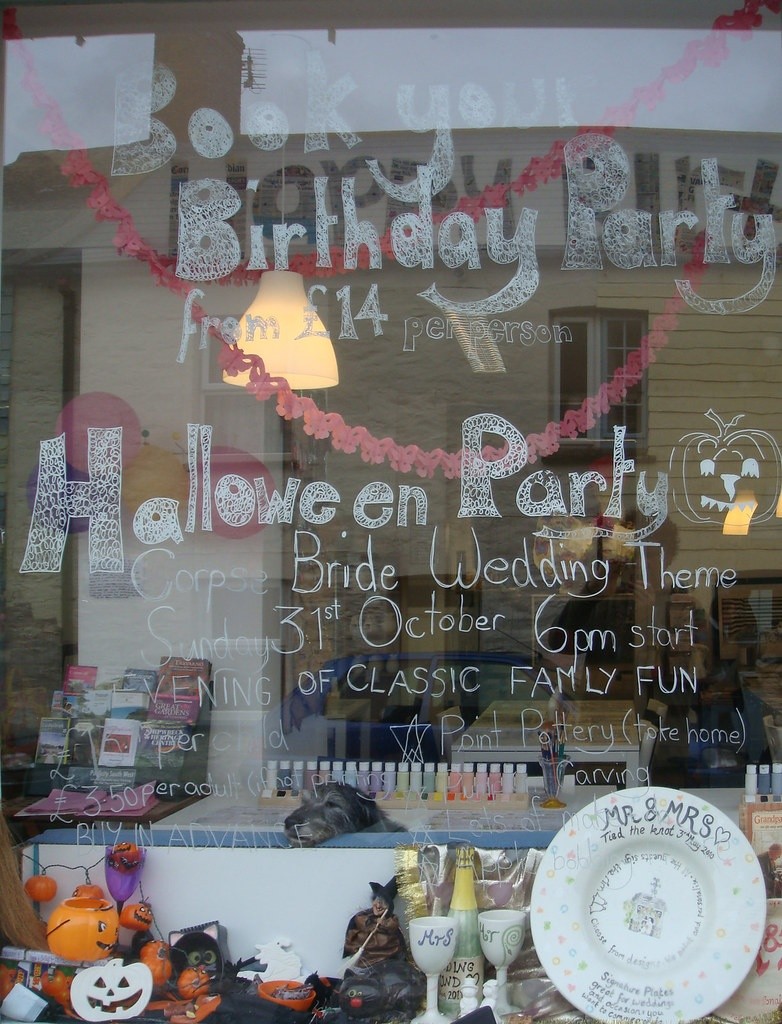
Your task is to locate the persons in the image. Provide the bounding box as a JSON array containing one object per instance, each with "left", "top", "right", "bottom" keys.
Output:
[
  {"left": 756, "top": 842, "right": 782, "bottom": 899},
  {"left": 342, "top": 876, "right": 408, "bottom": 963},
  {"left": 547, "top": 509, "right": 743, "bottom": 710}
]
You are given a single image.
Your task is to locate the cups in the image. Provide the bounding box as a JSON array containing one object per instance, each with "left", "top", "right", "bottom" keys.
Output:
[
  {"left": 0, "top": 983, "right": 48, "bottom": 1023},
  {"left": 537, "top": 753, "right": 571, "bottom": 809},
  {"left": 712, "top": 898, "right": 782, "bottom": 1024}
]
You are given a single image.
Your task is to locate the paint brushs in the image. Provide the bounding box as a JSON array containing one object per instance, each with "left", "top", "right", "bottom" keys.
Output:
[{"left": 537, "top": 707, "right": 567, "bottom": 798}]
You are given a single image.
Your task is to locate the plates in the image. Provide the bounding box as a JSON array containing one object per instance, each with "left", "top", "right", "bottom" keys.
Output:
[{"left": 529, "top": 786, "right": 768, "bottom": 1024}]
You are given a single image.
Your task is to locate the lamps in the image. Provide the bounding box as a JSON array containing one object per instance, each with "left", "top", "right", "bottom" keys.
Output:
[{"left": 221, "top": 135, "right": 339, "bottom": 393}]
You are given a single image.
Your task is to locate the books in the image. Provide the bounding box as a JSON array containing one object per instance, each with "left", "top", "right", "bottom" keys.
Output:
[
  {"left": 28, "top": 657, "right": 213, "bottom": 771},
  {"left": 736, "top": 800, "right": 782, "bottom": 900}
]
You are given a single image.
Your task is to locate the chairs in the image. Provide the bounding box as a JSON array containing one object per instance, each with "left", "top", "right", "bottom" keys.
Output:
[
  {"left": 430, "top": 705, "right": 466, "bottom": 770},
  {"left": 636, "top": 697, "right": 668, "bottom": 786}
]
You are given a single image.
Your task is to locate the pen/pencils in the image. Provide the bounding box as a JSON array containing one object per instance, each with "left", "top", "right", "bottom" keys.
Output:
[{"left": 82, "top": 799, "right": 107, "bottom": 811}]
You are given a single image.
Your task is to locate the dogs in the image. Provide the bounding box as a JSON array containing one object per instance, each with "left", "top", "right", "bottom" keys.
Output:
[{"left": 284, "top": 779, "right": 408, "bottom": 847}]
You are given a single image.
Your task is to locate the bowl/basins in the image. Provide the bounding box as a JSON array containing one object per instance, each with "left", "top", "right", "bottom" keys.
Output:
[{"left": 257, "top": 980, "right": 317, "bottom": 1012}]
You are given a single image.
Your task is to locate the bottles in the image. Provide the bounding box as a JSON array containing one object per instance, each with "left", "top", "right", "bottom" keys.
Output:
[
  {"left": 422, "top": 762, "right": 435, "bottom": 792},
  {"left": 464, "top": 762, "right": 501, "bottom": 794},
  {"left": 743, "top": 763, "right": 782, "bottom": 795},
  {"left": 345, "top": 761, "right": 357, "bottom": 788},
  {"left": 396, "top": 762, "right": 410, "bottom": 791},
  {"left": 319, "top": 761, "right": 330, "bottom": 786},
  {"left": 410, "top": 762, "right": 422, "bottom": 792},
  {"left": 436, "top": 762, "right": 448, "bottom": 793},
  {"left": 266, "top": 760, "right": 278, "bottom": 790},
  {"left": 450, "top": 763, "right": 461, "bottom": 793},
  {"left": 332, "top": 761, "right": 344, "bottom": 784},
  {"left": 305, "top": 761, "right": 318, "bottom": 790},
  {"left": 384, "top": 762, "right": 396, "bottom": 791},
  {"left": 370, "top": 761, "right": 383, "bottom": 791},
  {"left": 502, "top": 763, "right": 528, "bottom": 794},
  {"left": 439, "top": 843, "right": 485, "bottom": 1016},
  {"left": 357, "top": 762, "right": 369, "bottom": 792},
  {"left": 291, "top": 761, "right": 304, "bottom": 790},
  {"left": 278, "top": 760, "right": 291, "bottom": 790}
]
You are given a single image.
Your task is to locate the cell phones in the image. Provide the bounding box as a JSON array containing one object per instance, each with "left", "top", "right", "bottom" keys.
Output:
[{"left": 624, "top": 563, "right": 638, "bottom": 582}]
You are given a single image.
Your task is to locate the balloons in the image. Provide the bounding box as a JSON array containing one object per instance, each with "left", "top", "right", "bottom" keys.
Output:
[{"left": 24, "top": 392, "right": 276, "bottom": 540}]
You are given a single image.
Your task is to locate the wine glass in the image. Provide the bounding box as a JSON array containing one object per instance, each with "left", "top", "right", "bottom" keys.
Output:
[
  {"left": 477, "top": 909, "right": 527, "bottom": 1018},
  {"left": 408, "top": 916, "right": 458, "bottom": 1024}
]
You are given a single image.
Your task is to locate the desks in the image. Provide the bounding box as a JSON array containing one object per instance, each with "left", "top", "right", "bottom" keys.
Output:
[
  {"left": 450, "top": 699, "right": 640, "bottom": 788},
  {"left": 325, "top": 698, "right": 371, "bottom": 759},
  {"left": 1, "top": 787, "right": 212, "bottom": 836}
]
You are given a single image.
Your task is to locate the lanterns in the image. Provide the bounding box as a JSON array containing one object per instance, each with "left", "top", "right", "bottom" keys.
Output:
[
  {"left": 24, "top": 876, "right": 57, "bottom": 903},
  {"left": 46, "top": 897, "right": 119, "bottom": 962},
  {"left": 119, "top": 904, "right": 152, "bottom": 930},
  {"left": 140, "top": 940, "right": 172, "bottom": 988},
  {"left": 111, "top": 843, "right": 141, "bottom": 873},
  {"left": 72, "top": 884, "right": 103, "bottom": 900},
  {"left": 0, "top": 963, "right": 76, "bottom": 1008},
  {"left": 177, "top": 967, "right": 210, "bottom": 999}
]
